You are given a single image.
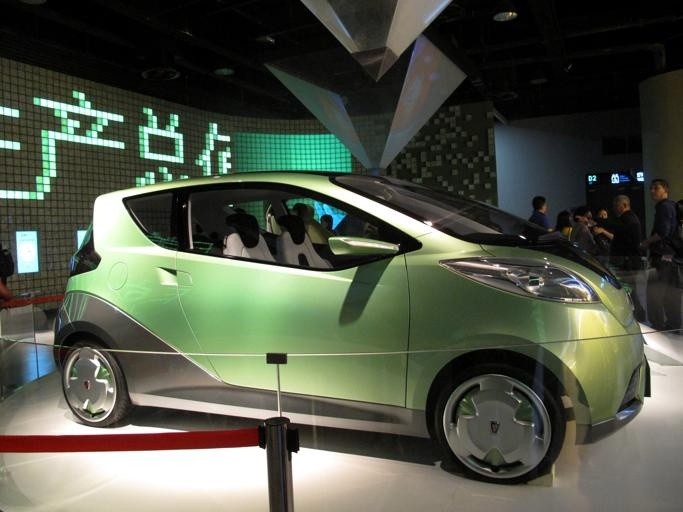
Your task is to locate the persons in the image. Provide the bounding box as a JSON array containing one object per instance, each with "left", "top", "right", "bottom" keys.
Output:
[
  {"left": 320, "top": 214, "right": 338, "bottom": 237},
  {"left": 529, "top": 195, "right": 615, "bottom": 271},
  {"left": 612, "top": 195, "right": 641, "bottom": 321},
  {"left": 0, "top": 279, "right": 13, "bottom": 300},
  {"left": 295, "top": 203, "right": 315, "bottom": 218},
  {"left": 641, "top": 178, "right": 683, "bottom": 334}
]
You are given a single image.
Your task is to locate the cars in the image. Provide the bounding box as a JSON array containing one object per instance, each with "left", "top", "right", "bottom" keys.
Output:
[{"left": 52, "top": 172, "right": 652, "bottom": 484}]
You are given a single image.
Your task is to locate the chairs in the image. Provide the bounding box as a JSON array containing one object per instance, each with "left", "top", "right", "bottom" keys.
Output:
[{"left": 221, "top": 204, "right": 335, "bottom": 272}]
